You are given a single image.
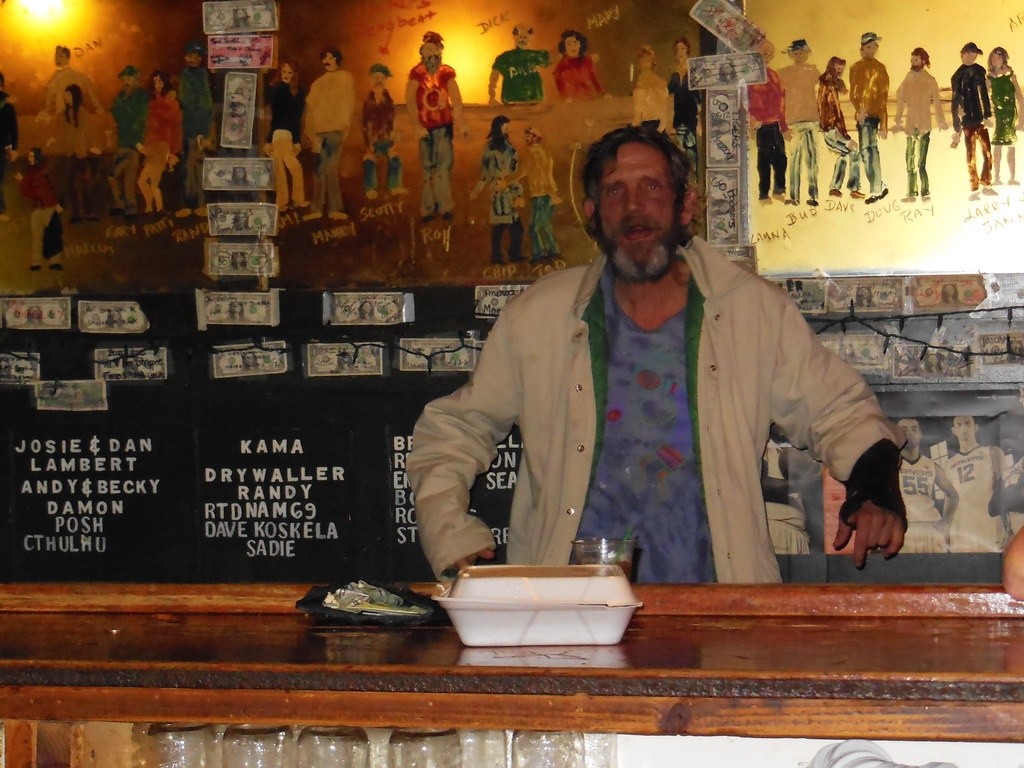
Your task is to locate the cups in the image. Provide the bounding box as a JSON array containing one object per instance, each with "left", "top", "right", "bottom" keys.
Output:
[
  {"left": 386, "top": 727, "right": 464, "bottom": 768},
  {"left": 147, "top": 722, "right": 215, "bottom": 768},
  {"left": 513, "top": 728, "right": 585, "bottom": 768},
  {"left": 295, "top": 727, "right": 369, "bottom": 767},
  {"left": 221, "top": 724, "right": 293, "bottom": 768},
  {"left": 571, "top": 537, "right": 637, "bottom": 584}
]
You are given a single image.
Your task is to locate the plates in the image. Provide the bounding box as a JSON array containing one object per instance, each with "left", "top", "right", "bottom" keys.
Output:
[{"left": 295, "top": 585, "right": 434, "bottom": 625}]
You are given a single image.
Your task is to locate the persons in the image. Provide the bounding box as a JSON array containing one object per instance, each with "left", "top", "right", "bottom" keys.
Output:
[
  {"left": 760, "top": 416, "right": 1023, "bottom": 553},
  {"left": 407, "top": 121, "right": 907, "bottom": 585},
  {"left": 1000, "top": 526, "right": 1024, "bottom": 600}
]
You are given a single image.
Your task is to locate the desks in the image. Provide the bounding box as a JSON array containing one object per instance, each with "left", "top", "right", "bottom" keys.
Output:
[{"left": 0, "top": 580, "right": 1024, "bottom": 768}]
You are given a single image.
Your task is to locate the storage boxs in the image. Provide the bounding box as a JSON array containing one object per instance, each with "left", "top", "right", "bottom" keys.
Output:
[{"left": 429, "top": 563, "right": 645, "bottom": 649}]
